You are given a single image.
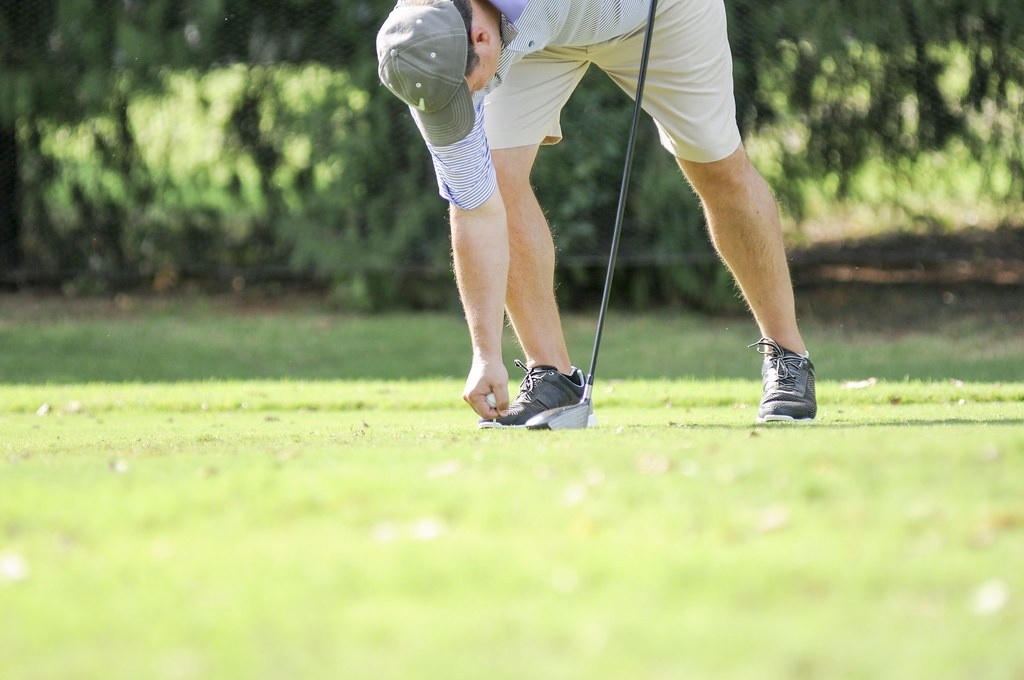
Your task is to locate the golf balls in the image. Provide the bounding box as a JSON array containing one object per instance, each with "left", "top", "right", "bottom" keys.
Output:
[{"left": 486, "top": 392, "right": 496, "bottom": 408}]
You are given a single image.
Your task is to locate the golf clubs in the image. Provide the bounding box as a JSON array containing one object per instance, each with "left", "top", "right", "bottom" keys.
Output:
[{"left": 525, "top": 0, "right": 659, "bottom": 431}]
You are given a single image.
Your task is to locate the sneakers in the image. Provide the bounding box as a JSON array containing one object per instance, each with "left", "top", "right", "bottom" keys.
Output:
[
  {"left": 747, "top": 336, "right": 817, "bottom": 423},
  {"left": 476, "top": 359, "right": 597, "bottom": 431}
]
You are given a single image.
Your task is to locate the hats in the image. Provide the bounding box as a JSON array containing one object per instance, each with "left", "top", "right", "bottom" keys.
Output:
[{"left": 375, "top": 0, "right": 474, "bottom": 146}]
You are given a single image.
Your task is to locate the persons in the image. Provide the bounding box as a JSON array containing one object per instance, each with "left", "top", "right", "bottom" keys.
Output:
[{"left": 375, "top": 0, "right": 818, "bottom": 433}]
